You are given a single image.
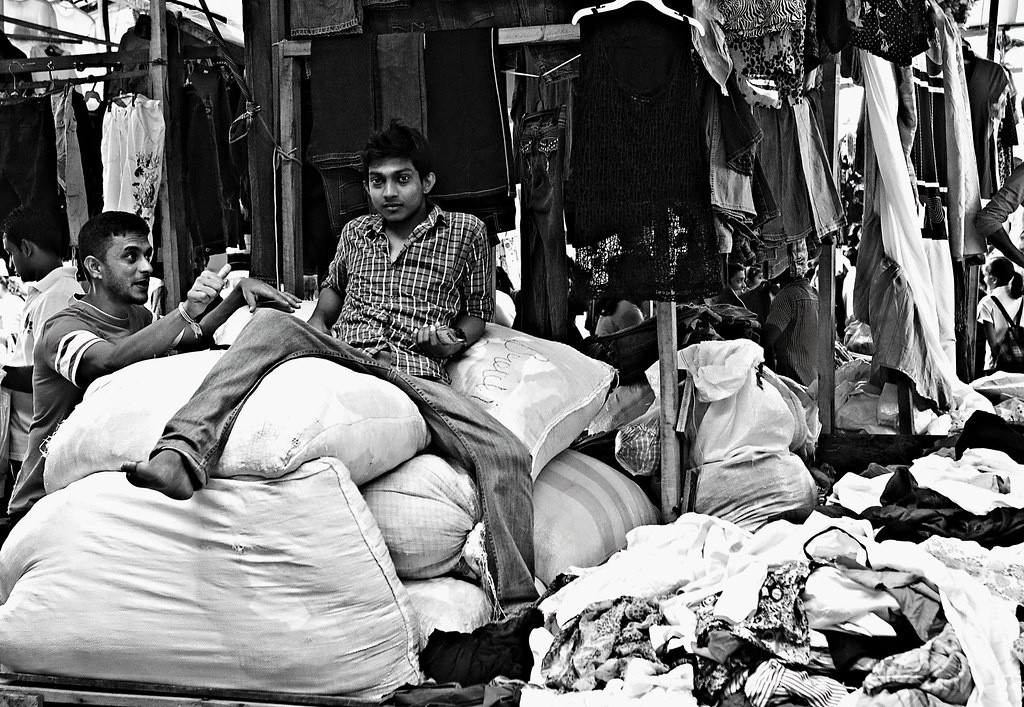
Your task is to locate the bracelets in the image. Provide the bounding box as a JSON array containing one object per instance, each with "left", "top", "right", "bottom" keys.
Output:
[
  {"left": 447, "top": 326, "right": 467, "bottom": 360},
  {"left": 178, "top": 302, "right": 202, "bottom": 338}
]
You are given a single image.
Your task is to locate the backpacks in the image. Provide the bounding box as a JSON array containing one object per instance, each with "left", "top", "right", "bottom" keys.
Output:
[{"left": 991, "top": 295, "right": 1024, "bottom": 374}]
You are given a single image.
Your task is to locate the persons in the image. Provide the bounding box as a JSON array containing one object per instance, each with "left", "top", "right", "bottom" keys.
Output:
[
  {"left": 592, "top": 284, "right": 643, "bottom": 335},
  {"left": 8, "top": 212, "right": 302, "bottom": 525},
  {"left": 809, "top": 244, "right": 873, "bottom": 357},
  {"left": 706, "top": 259, "right": 788, "bottom": 318},
  {"left": 761, "top": 263, "right": 818, "bottom": 384},
  {"left": 120, "top": 125, "right": 537, "bottom": 677},
  {"left": 975, "top": 164, "right": 1024, "bottom": 269},
  {"left": 0, "top": 205, "right": 85, "bottom": 481},
  {"left": 977, "top": 256, "right": 1024, "bottom": 371}
]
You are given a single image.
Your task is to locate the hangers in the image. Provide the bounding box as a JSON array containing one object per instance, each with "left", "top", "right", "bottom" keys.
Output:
[
  {"left": 571, "top": 0, "right": 706, "bottom": 37},
  {"left": 0, "top": 57, "right": 202, "bottom": 112}
]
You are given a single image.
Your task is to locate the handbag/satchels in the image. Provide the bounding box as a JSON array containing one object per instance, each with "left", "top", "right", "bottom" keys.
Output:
[{"left": 614, "top": 398, "right": 661, "bottom": 477}]
